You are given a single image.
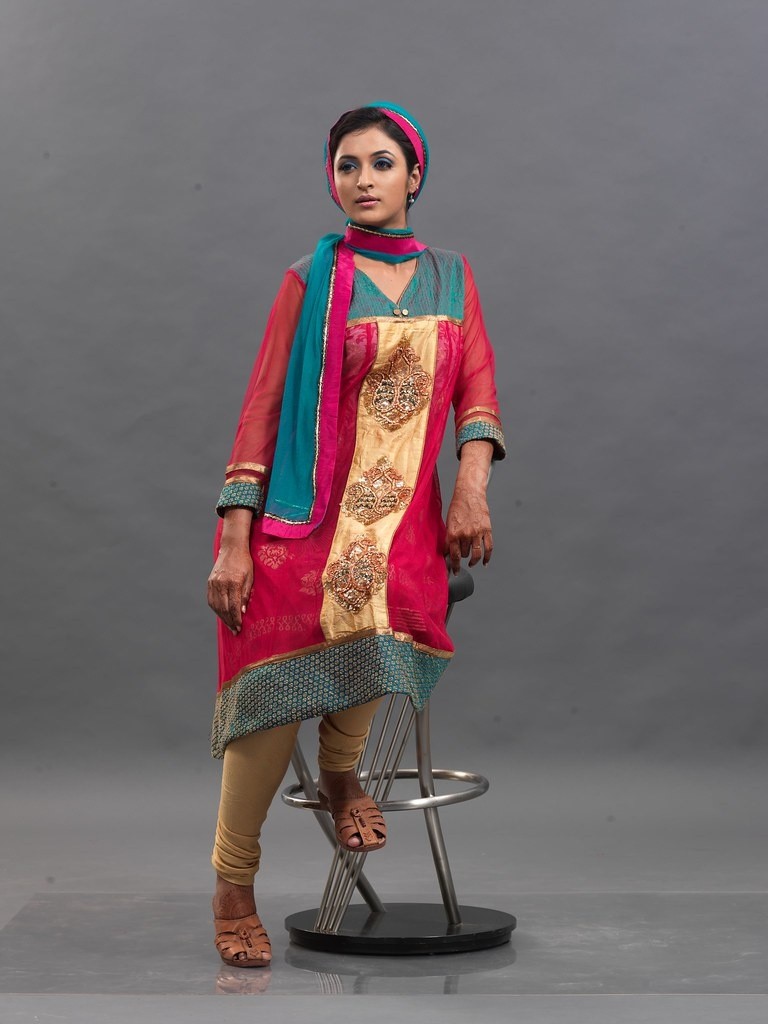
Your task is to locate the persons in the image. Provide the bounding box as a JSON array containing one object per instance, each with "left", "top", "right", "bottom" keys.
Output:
[{"left": 207, "top": 99, "right": 509, "bottom": 968}]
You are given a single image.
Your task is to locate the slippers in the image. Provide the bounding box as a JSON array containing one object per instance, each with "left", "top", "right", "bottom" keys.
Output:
[
  {"left": 213, "top": 901, "right": 272, "bottom": 968},
  {"left": 316, "top": 788, "right": 387, "bottom": 851}
]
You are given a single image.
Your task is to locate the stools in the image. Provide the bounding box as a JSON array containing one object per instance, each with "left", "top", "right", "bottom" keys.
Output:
[{"left": 279, "top": 562, "right": 519, "bottom": 957}]
